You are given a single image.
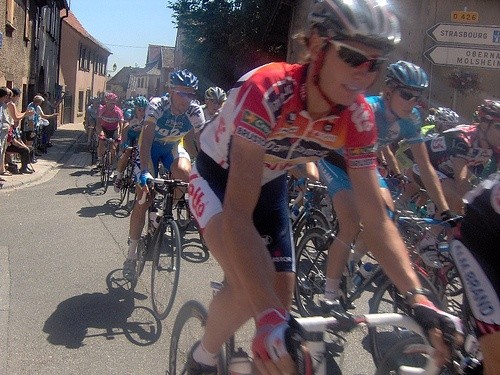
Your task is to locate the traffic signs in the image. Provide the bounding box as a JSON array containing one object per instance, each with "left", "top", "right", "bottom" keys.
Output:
[
  {"left": 423, "top": 45, "right": 499, "bottom": 71},
  {"left": 425, "top": 23, "right": 500, "bottom": 46}
]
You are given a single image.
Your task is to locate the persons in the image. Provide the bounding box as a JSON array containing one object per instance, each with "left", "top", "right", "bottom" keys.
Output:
[
  {"left": 84, "top": 62, "right": 500, "bottom": 375},
  {"left": 181, "top": 0, "right": 472, "bottom": 375},
  {"left": 0, "top": 87, "right": 61, "bottom": 184}
]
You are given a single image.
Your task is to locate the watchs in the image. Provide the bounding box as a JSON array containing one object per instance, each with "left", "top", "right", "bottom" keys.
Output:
[{"left": 400, "top": 287, "right": 433, "bottom": 301}]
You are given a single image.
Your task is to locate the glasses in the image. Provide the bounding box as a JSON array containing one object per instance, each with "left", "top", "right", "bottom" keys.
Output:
[
  {"left": 393, "top": 86, "right": 422, "bottom": 103},
  {"left": 319, "top": 36, "right": 388, "bottom": 72},
  {"left": 485, "top": 122, "right": 500, "bottom": 130},
  {"left": 174, "top": 91, "right": 195, "bottom": 99},
  {"left": 207, "top": 100, "right": 224, "bottom": 105}
]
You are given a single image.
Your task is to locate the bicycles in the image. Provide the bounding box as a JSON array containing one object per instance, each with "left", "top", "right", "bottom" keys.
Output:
[{"left": 82, "top": 119, "right": 484, "bottom": 375}]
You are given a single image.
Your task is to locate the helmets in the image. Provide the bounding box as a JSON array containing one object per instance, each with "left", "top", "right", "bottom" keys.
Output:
[
  {"left": 169, "top": 69, "right": 198, "bottom": 91},
  {"left": 477, "top": 99, "right": 500, "bottom": 121},
  {"left": 307, "top": 0, "right": 401, "bottom": 53},
  {"left": 92, "top": 96, "right": 100, "bottom": 103},
  {"left": 105, "top": 93, "right": 118, "bottom": 103},
  {"left": 135, "top": 96, "right": 149, "bottom": 107},
  {"left": 205, "top": 86, "right": 227, "bottom": 101},
  {"left": 384, "top": 60, "right": 429, "bottom": 89},
  {"left": 428, "top": 106, "right": 460, "bottom": 130},
  {"left": 126, "top": 101, "right": 134, "bottom": 107}
]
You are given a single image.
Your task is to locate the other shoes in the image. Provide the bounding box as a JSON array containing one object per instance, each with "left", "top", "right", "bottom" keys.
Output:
[
  {"left": 96, "top": 159, "right": 103, "bottom": 168},
  {"left": 0, "top": 142, "right": 52, "bottom": 190},
  {"left": 320, "top": 297, "right": 346, "bottom": 315},
  {"left": 86, "top": 141, "right": 91, "bottom": 146},
  {"left": 187, "top": 340, "right": 217, "bottom": 375},
  {"left": 114, "top": 178, "right": 121, "bottom": 193},
  {"left": 122, "top": 257, "right": 136, "bottom": 280},
  {"left": 130, "top": 178, "right": 136, "bottom": 190}
]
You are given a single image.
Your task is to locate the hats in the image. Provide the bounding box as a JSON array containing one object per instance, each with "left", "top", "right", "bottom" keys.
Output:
[{"left": 34, "top": 95, "right": 45, "bottom": 103}]
